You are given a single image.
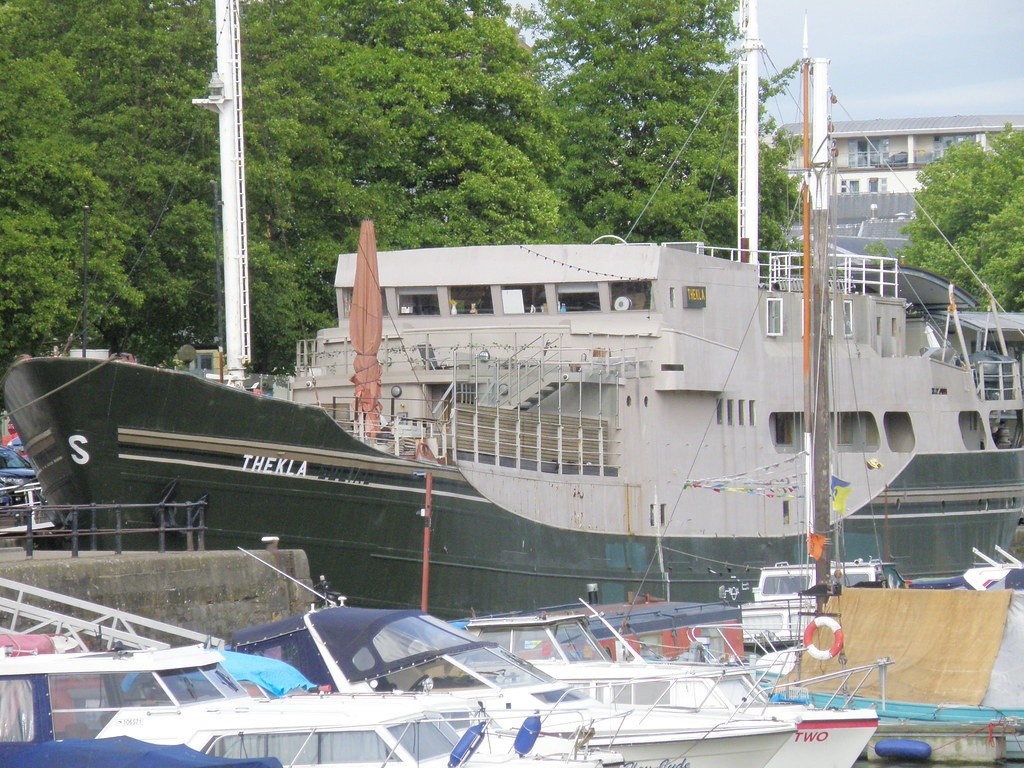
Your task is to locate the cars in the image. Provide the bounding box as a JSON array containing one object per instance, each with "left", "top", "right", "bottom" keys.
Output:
[{"left": 1, "top": 446, "right": 48, "bottom": 506}]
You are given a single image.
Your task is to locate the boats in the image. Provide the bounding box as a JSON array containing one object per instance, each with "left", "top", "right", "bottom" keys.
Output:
[{"left": 2, "top": 1, "right": 1024, "bottom": 768}]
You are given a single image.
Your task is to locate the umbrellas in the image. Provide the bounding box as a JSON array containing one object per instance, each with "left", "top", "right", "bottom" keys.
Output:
[{"left": 349, "top": 219, "right": 382, "bottom": 440}]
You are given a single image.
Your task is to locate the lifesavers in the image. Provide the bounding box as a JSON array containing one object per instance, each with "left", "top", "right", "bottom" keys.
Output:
[{"left": 804, "top": 616, "right": 844, "bottom": 661}]
